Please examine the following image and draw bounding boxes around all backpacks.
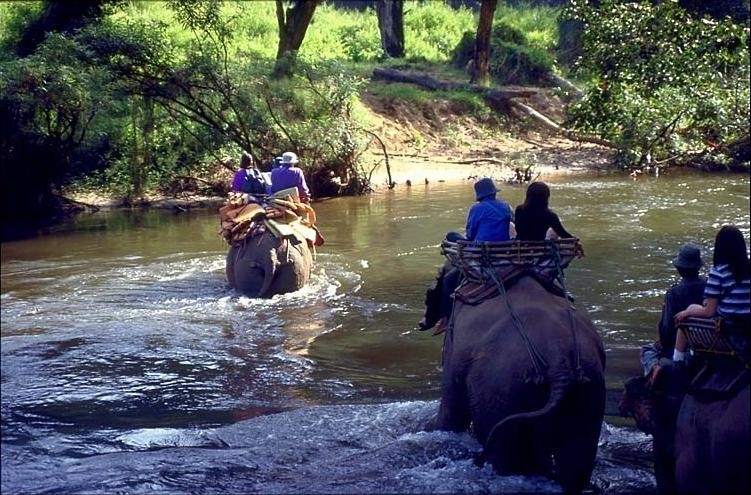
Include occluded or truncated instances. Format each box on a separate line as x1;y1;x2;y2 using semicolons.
243;167;267;195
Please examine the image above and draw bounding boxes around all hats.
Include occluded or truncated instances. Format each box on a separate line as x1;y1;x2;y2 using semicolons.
271;155;283;168
280;152;300;165
672;244;705;270
472;177;501;201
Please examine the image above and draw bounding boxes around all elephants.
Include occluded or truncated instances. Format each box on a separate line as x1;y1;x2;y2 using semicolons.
618;354;751;494
435;275;608;495
225;220;311;299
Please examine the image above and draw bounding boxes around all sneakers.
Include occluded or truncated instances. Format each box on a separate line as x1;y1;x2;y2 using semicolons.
648;364;667;400
670;359;688;378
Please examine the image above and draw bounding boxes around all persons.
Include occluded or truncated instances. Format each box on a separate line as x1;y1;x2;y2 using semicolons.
512;181;588;303
417;176;511;336
266;152;312;205
272;156;283;168
639;243;709;397
232;152;265;194
657;225;750;372
